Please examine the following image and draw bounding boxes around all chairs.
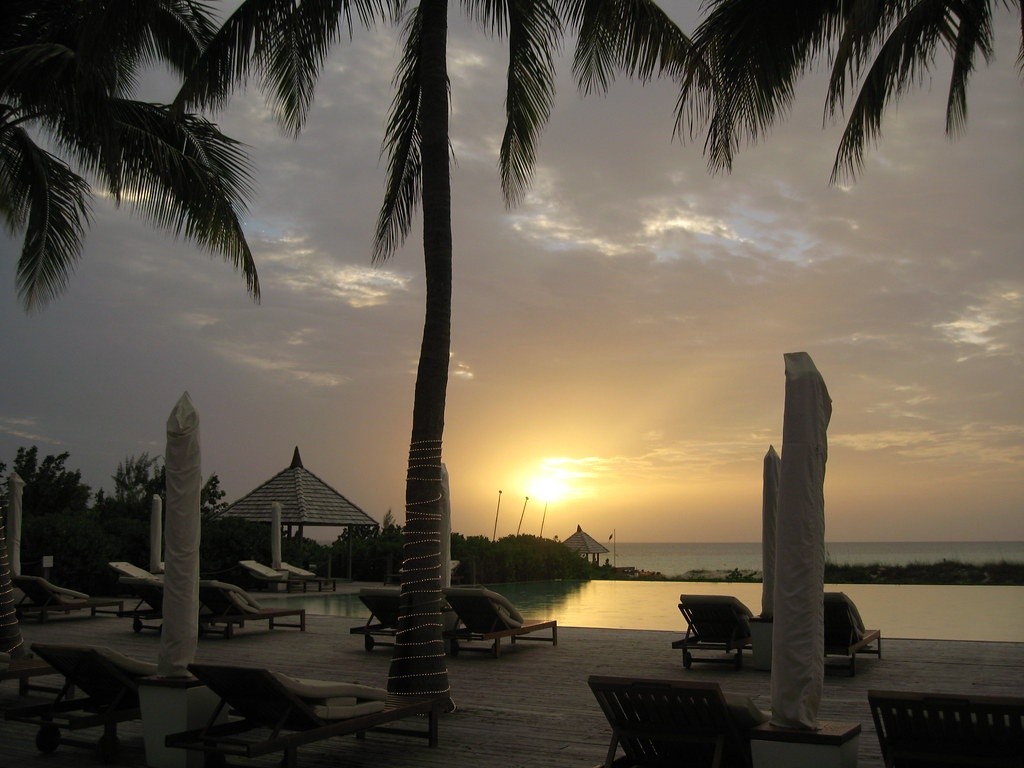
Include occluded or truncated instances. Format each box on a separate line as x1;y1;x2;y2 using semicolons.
239;560;306;593
118;577;162;634
4;643;159;761
672;594;754;668
165;664;438;768
825;592;882;676
349;589;466;656
199;580;306;638
13;576;124;623
587;675;772;768
867;689;1024;768
281;562;336;592
442;588;557;657
